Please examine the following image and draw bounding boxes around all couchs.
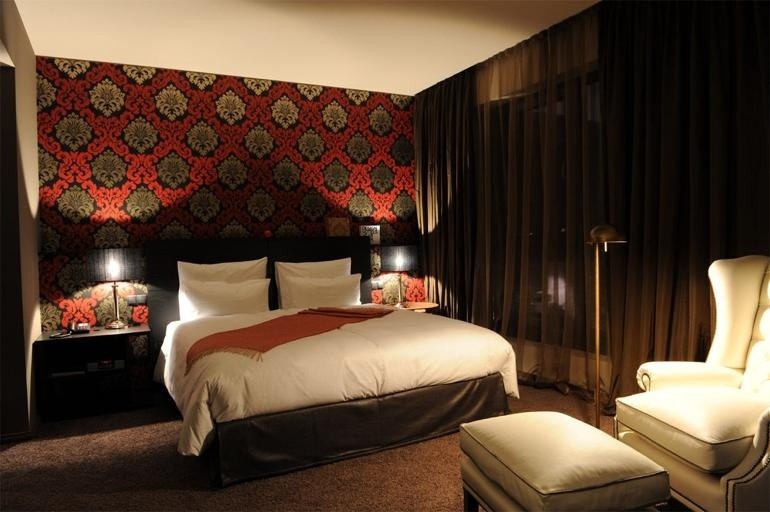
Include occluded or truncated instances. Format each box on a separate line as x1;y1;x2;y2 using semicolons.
611;252;770;512
456;410;672;512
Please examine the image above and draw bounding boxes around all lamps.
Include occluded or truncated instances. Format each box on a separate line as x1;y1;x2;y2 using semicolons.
378;244;422;309
584;223;631;431
85;247;149;329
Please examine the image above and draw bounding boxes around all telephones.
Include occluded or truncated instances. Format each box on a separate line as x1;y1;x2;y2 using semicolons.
67;321;90;334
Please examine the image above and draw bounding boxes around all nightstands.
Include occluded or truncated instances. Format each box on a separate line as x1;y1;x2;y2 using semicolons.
32;324;152;414
385;301;439;313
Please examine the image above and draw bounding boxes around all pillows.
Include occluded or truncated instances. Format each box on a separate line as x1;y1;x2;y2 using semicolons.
281;273;363;312
175;255;269;290
178;277;272;322
273;257;352;310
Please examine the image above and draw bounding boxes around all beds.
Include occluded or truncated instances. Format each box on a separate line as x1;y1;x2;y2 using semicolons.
146;235;523;491
